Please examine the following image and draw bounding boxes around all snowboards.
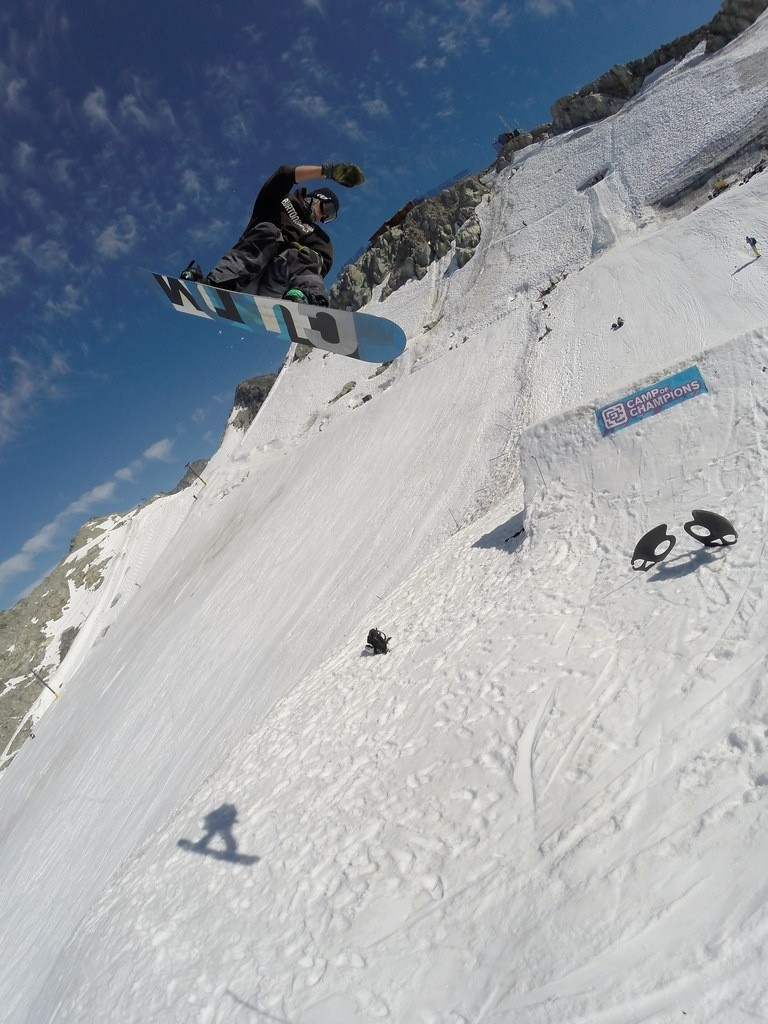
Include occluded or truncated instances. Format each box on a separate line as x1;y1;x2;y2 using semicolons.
136;265;408;367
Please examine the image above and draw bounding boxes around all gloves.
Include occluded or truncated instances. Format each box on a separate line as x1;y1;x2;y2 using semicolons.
321;162;365;188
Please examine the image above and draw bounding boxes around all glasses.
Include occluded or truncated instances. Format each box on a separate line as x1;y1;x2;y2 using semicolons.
320;201;337;224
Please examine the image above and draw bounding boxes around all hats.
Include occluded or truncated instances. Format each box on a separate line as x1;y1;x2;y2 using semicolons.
305;187;340;211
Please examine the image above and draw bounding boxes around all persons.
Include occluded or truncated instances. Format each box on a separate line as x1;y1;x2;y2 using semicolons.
180;162;365;308
612;317;624;328
746;236;761;259
